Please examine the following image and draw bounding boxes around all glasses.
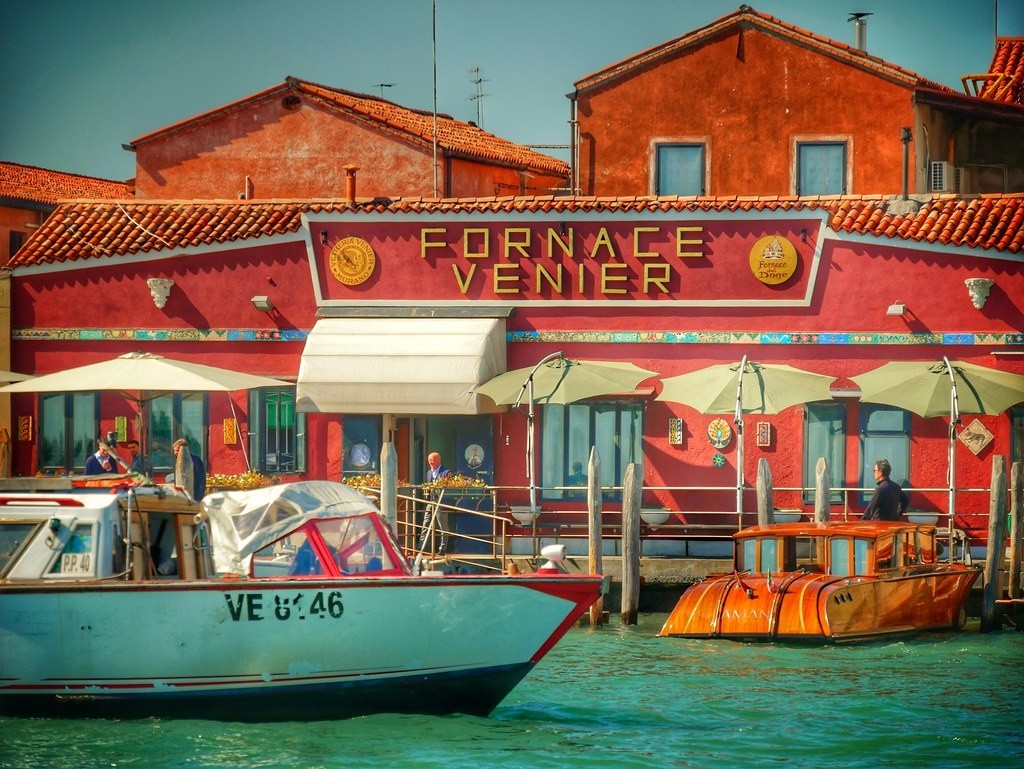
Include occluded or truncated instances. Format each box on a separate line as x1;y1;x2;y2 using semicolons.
873;469;879;472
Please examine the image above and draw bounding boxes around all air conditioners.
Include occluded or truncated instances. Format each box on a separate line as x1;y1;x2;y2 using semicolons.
954;167;973;194
932;161;955;194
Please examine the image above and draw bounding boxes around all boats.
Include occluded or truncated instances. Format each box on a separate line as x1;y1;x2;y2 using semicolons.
2;480;614;720
660;521;987;646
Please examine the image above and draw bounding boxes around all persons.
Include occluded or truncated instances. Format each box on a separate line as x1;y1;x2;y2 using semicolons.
417;453;453;557
171;437;205;501
859;459;909;522
86;440;154;478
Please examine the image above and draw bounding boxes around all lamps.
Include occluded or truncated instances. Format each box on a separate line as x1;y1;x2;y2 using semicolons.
252;296;273;312
887;299;906;318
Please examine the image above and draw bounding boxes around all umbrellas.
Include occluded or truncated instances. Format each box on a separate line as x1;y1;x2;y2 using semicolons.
473;354;659;557
655;358;839;512
846;358;1024;531
0;356;295;472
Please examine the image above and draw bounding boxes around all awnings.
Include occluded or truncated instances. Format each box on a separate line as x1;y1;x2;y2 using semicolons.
291;315;506;415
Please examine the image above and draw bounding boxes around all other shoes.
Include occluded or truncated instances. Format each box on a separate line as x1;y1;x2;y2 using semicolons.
408;548;424;555
437;550;445;555
